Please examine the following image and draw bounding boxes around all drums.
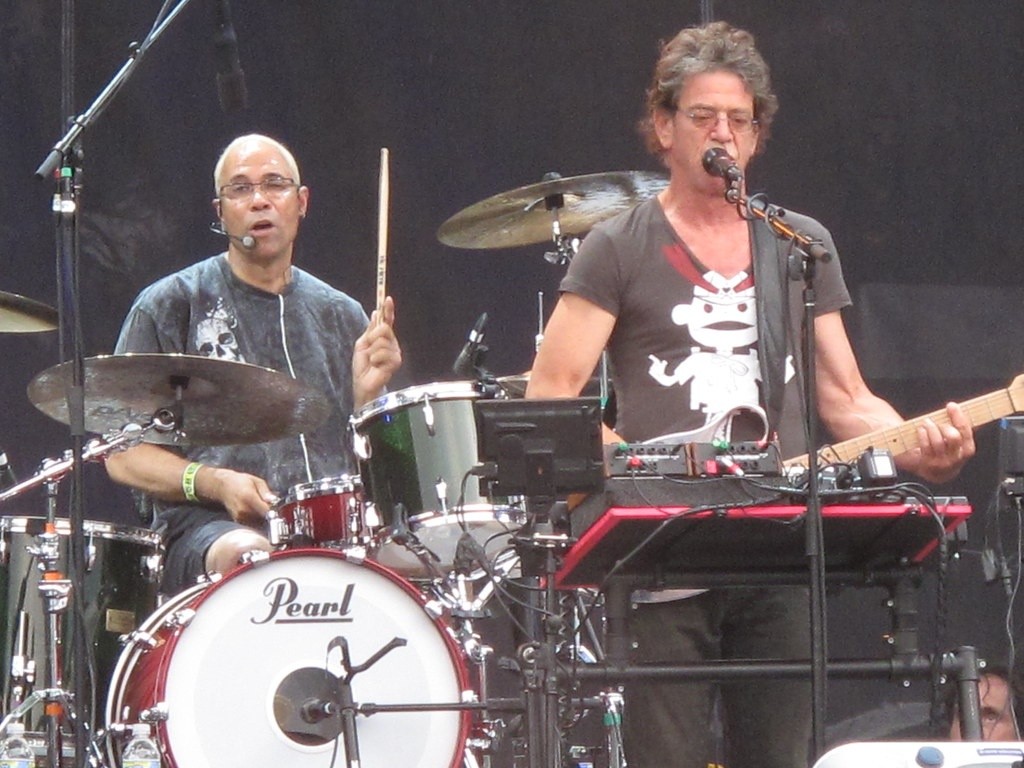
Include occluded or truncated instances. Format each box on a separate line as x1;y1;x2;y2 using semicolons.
263;474;377;551
101;546;481;768
344;368;535;573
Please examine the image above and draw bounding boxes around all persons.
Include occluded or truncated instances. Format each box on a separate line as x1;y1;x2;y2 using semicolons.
527;23;976;768
100;134;402;594
941;664;1024;743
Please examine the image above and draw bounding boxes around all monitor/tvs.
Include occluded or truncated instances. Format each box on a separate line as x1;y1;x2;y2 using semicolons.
475;399;604;496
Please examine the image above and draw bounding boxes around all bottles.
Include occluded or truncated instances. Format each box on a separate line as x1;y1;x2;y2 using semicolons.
122;724;160;768
0;723;35;768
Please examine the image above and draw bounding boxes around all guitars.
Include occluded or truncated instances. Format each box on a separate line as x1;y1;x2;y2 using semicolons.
553;370;1024;522
1;513;168;759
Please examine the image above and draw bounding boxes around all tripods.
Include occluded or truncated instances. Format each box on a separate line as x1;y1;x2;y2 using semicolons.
0;380;184;768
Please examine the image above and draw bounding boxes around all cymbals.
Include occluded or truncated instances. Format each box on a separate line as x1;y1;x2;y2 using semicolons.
436;168;671;253
1;288;68;338
25;351;330;447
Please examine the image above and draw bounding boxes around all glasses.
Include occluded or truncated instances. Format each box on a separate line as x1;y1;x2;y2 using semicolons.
218;177;300;200
671;105;760;132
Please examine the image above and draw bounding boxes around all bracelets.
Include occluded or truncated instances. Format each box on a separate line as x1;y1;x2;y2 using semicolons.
181;462;207;505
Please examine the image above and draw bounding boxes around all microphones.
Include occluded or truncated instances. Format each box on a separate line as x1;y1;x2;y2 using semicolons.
209;222;255;248
204;0;249;114
299;697;336;723
453;312;488;377
702;148;742;181
